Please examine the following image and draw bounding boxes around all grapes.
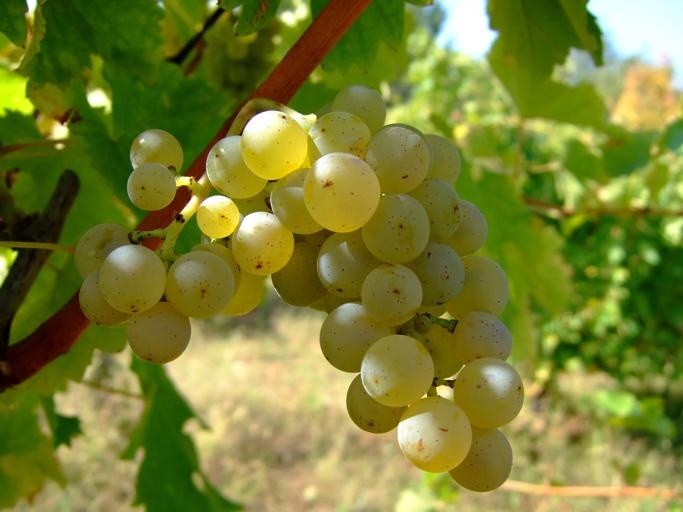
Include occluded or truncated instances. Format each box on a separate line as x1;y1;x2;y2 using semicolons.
74;83;525;493
